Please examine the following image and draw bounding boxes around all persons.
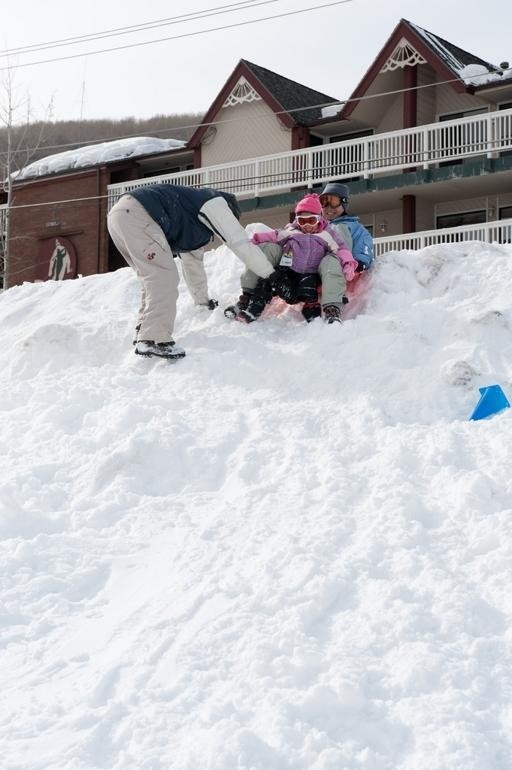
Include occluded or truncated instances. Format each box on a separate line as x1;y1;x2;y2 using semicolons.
106;182;279;361
224;181;376;324
235;192;358;324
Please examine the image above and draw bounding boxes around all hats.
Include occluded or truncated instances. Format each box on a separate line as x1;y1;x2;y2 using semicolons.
295;192;322;215
319;182;350;199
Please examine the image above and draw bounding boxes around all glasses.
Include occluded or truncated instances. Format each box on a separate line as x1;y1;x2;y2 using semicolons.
319;197;349;209
295;213;321;227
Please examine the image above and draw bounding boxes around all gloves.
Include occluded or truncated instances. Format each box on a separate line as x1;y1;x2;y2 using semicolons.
341;263;356;282
266;269;297;305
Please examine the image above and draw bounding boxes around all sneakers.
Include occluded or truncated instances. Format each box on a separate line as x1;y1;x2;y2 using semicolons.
132;324;186;360
223;293;266;325
301;302;344;326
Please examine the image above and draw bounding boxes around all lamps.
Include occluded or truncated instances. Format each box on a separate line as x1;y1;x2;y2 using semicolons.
380;219;389;233
487;204;495;216
